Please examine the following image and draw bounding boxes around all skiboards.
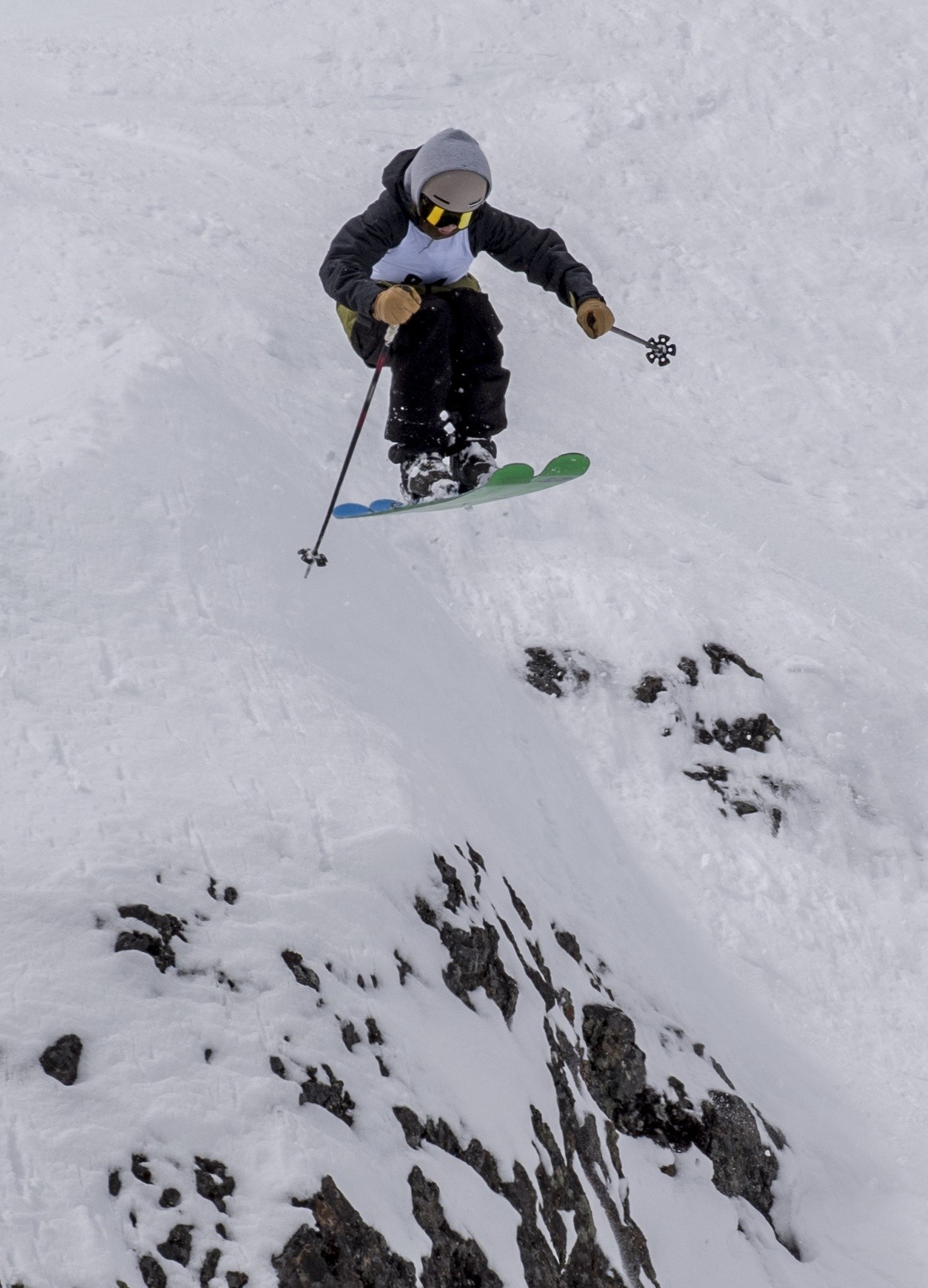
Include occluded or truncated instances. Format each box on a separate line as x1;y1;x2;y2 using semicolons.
331;452;592;521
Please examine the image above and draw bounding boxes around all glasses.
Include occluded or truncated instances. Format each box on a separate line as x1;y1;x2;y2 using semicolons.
419;199;477;230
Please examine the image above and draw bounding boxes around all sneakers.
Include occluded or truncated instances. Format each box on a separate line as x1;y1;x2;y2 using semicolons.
400;448;460;506
452;441;498;493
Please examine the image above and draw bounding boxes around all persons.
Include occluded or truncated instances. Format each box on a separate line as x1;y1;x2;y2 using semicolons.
319;127;615;503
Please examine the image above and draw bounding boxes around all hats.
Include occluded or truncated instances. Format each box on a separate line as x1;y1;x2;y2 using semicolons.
420;169;489;211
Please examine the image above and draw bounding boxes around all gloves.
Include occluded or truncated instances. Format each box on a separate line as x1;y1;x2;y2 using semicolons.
371;284;421;325
576;297;614;339
